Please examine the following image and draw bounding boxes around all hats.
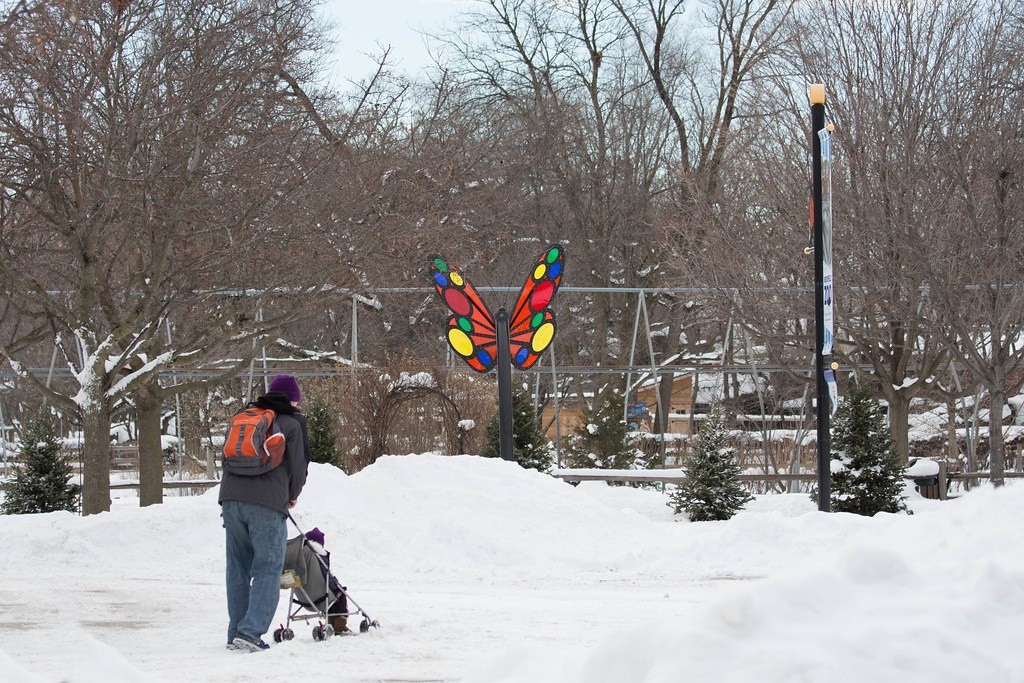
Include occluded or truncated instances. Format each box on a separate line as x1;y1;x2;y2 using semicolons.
306;527;325;546
268;374;300;402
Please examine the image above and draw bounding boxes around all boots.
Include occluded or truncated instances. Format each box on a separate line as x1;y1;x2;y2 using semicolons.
328;616;333;625
333;616;352;636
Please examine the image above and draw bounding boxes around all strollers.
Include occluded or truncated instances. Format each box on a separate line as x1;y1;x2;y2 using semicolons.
274;504;381;644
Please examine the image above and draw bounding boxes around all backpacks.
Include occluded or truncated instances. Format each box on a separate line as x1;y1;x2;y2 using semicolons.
221;404;286;476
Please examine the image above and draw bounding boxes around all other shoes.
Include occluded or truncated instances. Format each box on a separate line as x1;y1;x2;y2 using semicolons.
233;631;270;652
226;640;239;650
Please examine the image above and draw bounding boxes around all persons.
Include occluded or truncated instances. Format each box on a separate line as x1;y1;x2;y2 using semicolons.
217;374;311;652
305;527;358;636
